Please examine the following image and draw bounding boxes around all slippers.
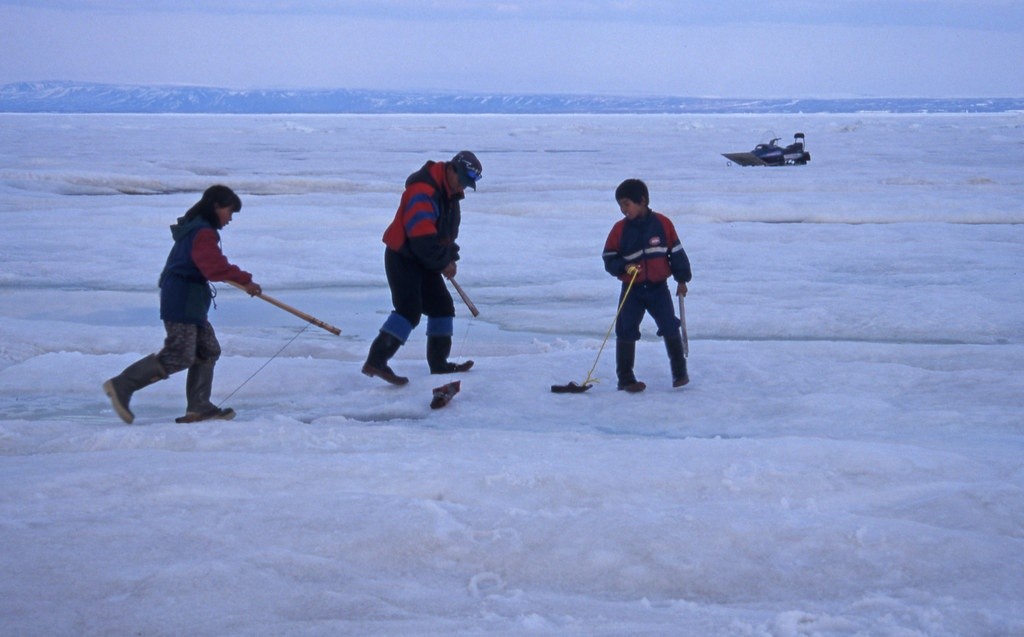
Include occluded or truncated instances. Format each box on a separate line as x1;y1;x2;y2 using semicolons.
431;381;461;410
551;381;592;394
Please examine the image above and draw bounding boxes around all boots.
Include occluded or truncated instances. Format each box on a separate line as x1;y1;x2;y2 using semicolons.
662;329;689;387
104;353;169;424
426;316;474;374
616;337;646;392
362;311;409;385
186;357;237;422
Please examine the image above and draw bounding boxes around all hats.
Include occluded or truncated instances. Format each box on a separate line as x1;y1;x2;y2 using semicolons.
452;151;484;191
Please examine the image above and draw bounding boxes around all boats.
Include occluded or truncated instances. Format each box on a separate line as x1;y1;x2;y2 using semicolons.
720;138;810;167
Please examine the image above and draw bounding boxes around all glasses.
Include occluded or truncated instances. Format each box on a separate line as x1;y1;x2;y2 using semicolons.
455;154;483;182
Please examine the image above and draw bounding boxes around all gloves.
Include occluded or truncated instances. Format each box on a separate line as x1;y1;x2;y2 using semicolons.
246;283;262;297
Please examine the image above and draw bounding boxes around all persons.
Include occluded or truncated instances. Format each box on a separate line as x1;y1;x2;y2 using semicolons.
362;149;484;386
103;185;263;423
602;178;692;392
